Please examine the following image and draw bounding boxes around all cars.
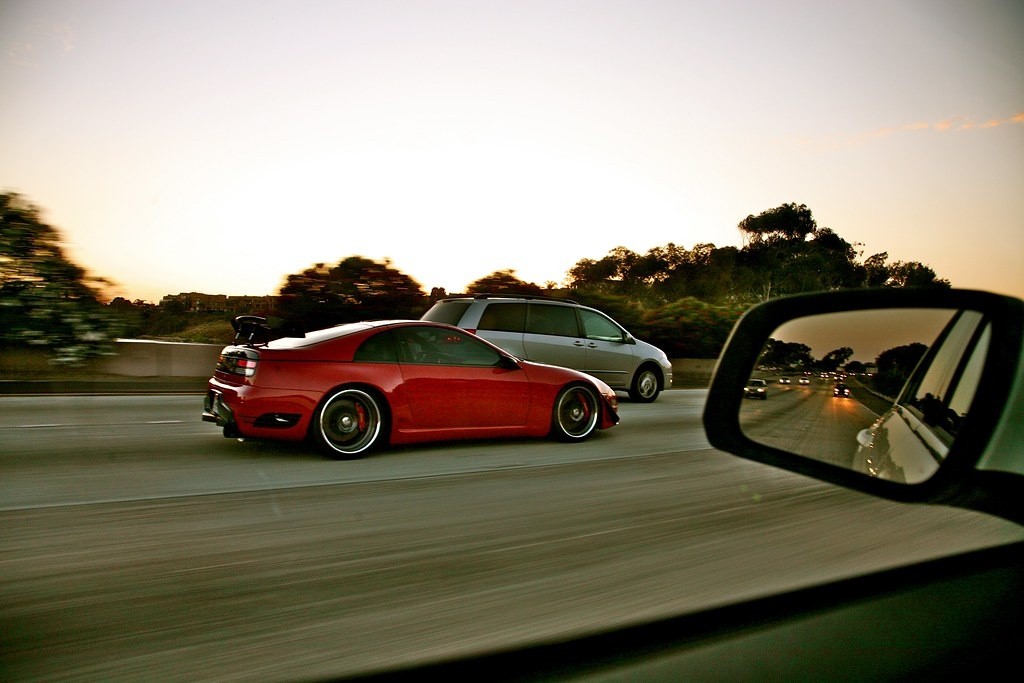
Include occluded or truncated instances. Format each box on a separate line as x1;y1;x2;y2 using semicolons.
739;368;876;408
199;313;620;455
414;294;676;405
